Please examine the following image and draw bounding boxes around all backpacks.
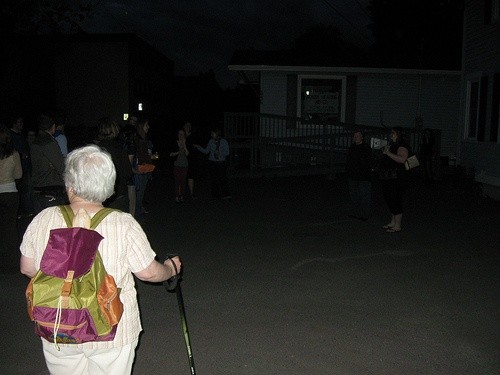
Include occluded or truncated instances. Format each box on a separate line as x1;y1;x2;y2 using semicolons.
25;204;123;344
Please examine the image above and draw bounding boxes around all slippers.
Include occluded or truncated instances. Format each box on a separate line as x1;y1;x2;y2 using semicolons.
384;223;405;235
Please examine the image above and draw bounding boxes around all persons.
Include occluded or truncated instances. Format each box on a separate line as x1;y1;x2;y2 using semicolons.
0;115;231;273
19;145;182;375
345;126;436;232
